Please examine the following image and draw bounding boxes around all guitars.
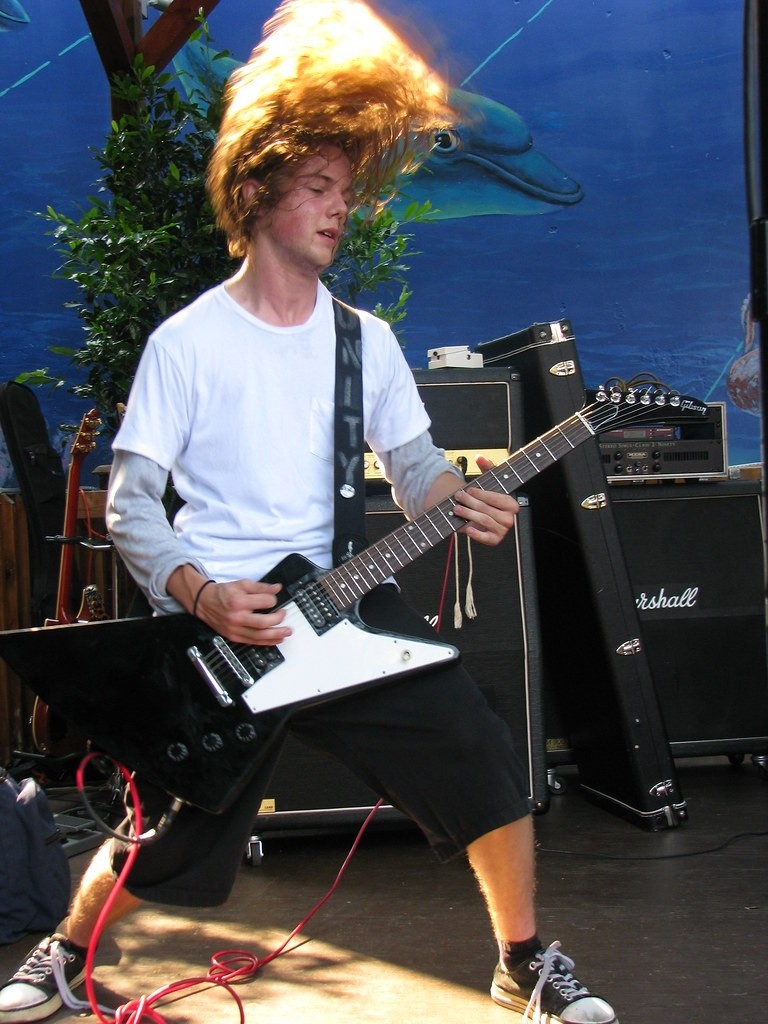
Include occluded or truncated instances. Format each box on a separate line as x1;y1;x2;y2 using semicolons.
0;386;715;822
30;404;102;753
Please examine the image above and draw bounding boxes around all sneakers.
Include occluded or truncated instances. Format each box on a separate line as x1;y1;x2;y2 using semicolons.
0;936;87;1024
491;941;620;1024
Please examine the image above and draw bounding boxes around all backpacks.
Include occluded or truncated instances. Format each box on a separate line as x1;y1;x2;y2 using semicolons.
1;767;71;942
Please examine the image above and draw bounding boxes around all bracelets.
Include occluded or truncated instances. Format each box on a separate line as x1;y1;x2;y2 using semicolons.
193;579;218;616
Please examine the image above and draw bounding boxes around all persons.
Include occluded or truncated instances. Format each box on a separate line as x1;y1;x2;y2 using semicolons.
0;38;627;1024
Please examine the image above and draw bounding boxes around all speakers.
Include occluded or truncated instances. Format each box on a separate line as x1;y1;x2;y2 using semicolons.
250;491;548;833
608;480;768;755
